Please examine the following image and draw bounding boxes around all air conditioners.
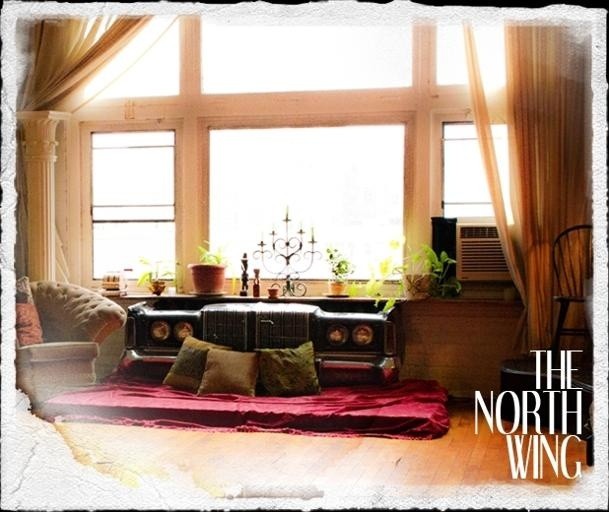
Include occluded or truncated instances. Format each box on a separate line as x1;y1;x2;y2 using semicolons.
455;215;516;300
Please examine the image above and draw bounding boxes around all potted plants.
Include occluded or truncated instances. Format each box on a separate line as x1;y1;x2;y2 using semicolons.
136;256;177;295
324;244;352;298
370;236;463;313
187;238;230;297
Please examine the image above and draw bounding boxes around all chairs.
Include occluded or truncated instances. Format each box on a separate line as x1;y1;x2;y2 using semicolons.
499;222;593;467
16;281;129;406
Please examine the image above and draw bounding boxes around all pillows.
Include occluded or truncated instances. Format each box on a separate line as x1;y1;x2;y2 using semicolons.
16;276;43;346
197;347;260;397
162;335;233;393
252;340;322;397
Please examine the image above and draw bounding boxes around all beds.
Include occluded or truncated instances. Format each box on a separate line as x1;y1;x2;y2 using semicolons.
42;302;451;440
